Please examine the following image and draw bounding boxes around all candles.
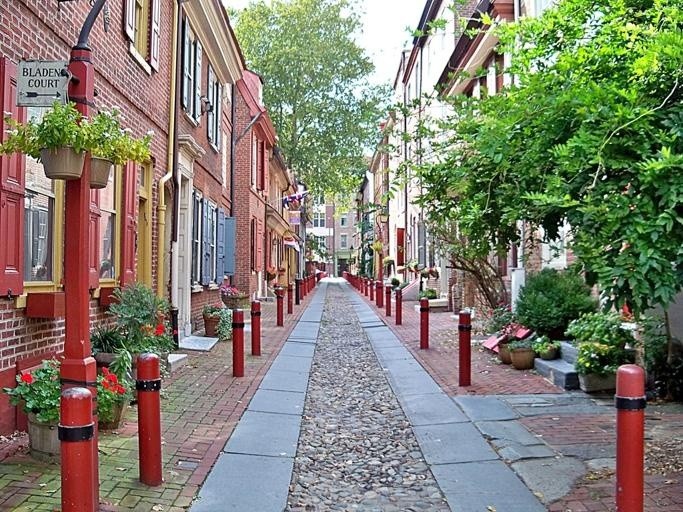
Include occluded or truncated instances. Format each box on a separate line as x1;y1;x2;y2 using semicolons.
0;99;91;181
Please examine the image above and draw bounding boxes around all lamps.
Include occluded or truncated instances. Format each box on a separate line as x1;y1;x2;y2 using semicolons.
376;206;390;237
197;95;213;119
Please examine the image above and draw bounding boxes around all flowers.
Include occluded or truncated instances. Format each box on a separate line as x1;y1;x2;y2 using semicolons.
2;354;65;425
481;304;518;343
574;341;633;378
139;306;178;353
217;284;239;296
96;366;136;423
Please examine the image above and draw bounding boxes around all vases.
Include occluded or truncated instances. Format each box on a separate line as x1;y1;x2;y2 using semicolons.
498;341;513;364
221;294;250;309
26;411;62;465
153;350;169;372
577;373;616;392
98;396;124;430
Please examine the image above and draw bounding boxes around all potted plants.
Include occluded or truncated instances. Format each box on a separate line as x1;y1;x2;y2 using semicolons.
203;303;234;341
534;335;560;360
88;319;139;384
88;105;154;189
508;339;536;370
